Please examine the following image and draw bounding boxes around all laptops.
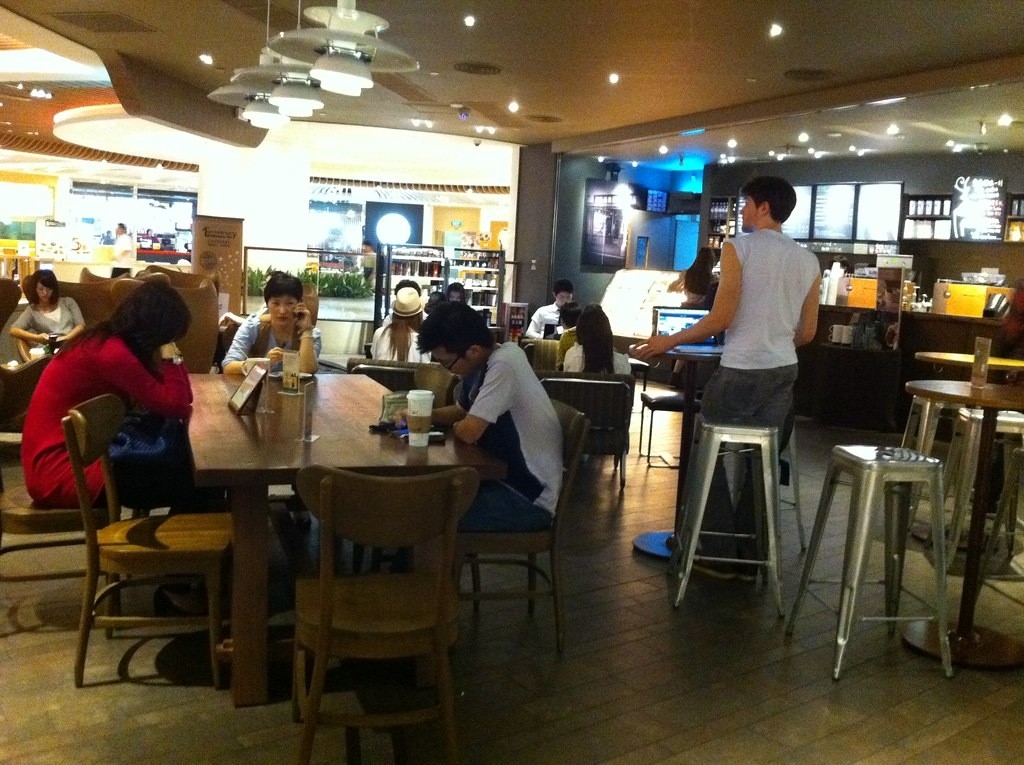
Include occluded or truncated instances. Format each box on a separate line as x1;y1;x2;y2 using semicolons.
653;306;725;353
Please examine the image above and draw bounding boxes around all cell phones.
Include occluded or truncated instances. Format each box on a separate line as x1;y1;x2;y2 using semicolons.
396;432;443;442
49;334;58;341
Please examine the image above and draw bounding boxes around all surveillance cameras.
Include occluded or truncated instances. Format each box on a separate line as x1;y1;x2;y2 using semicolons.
474;140;481;146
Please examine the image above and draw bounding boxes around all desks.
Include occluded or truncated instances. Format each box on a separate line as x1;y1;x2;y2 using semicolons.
182;372;507;707
814;341;901;433
911;352;1024;552
901;379;1024;672
629;342;725;558
629;357;650;456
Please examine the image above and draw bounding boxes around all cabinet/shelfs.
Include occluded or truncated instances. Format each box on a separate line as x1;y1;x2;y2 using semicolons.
897;194;1024;243
706;196;738;269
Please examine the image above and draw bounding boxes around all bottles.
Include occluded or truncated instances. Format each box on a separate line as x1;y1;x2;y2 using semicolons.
455;251;499;268
511;309;524;325
718;203;728;220
464;289;496;306
855;314;897;350
477;309;491;327
711;203;718;220
391;260;405;275
509;329;523;344
713;221;720;234
406;260;440;277
421;280;443;299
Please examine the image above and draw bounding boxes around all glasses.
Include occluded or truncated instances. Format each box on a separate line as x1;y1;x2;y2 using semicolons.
432;343;476;372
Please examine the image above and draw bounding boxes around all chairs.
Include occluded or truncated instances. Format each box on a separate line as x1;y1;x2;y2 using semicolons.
0;264;636;765
639;357;703;469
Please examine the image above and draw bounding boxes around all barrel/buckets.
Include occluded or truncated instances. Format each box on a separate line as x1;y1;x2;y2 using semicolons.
984;294;1011;318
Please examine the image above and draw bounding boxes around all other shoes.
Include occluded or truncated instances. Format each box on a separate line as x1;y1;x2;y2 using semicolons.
738;559;759;580
692;548;736;579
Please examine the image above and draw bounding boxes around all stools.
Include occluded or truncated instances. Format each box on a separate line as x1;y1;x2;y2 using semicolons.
901;397;1024;580
786;443;954;683
666;413;783;617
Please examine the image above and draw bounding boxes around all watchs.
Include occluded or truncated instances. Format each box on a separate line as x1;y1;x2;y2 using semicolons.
162;354;182;366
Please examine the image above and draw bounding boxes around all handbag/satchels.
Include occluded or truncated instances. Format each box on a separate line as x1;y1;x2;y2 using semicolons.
109;410;191;509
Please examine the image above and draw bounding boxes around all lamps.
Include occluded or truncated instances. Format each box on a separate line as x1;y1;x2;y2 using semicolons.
206;0;420;130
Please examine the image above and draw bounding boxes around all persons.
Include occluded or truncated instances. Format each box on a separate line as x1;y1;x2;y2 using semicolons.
145;229;160;243
369;279;468;365
21;279;232;619
666;246;719;388
111;223;136;278
555;302;582;370
220;271;319;532
526;278;574;339
392;299;561;575
10;268;86;351
564;301;633;377
633;178;821;580
360;241;376;284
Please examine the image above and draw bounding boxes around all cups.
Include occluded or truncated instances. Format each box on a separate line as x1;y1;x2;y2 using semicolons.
449;278;472;287
30;348;45;360
828;324;853;347
242;358;270;388
473;280;497;287
406;390;434;446
393;248;443;258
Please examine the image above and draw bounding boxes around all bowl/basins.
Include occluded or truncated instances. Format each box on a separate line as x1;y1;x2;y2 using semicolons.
854;263;868;275
962;273;1005;287
864;268;878;278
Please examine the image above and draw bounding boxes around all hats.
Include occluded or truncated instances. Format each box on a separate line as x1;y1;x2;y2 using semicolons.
391;287;425;316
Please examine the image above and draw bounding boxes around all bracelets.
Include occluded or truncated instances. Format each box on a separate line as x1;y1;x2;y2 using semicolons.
297;325;314;335
299;335;315;341
